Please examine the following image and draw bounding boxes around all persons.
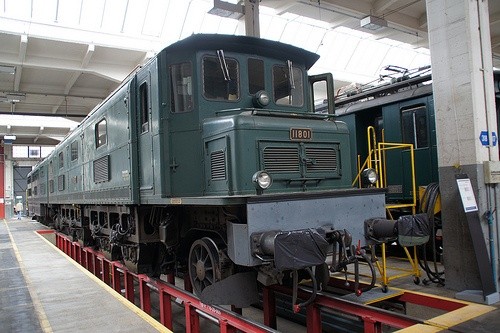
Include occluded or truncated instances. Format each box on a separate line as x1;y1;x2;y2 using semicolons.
15;200;23;220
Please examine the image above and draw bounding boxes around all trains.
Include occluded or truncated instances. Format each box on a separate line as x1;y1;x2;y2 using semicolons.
352;300;390;307
24;32;343;299
312;65;443;262
